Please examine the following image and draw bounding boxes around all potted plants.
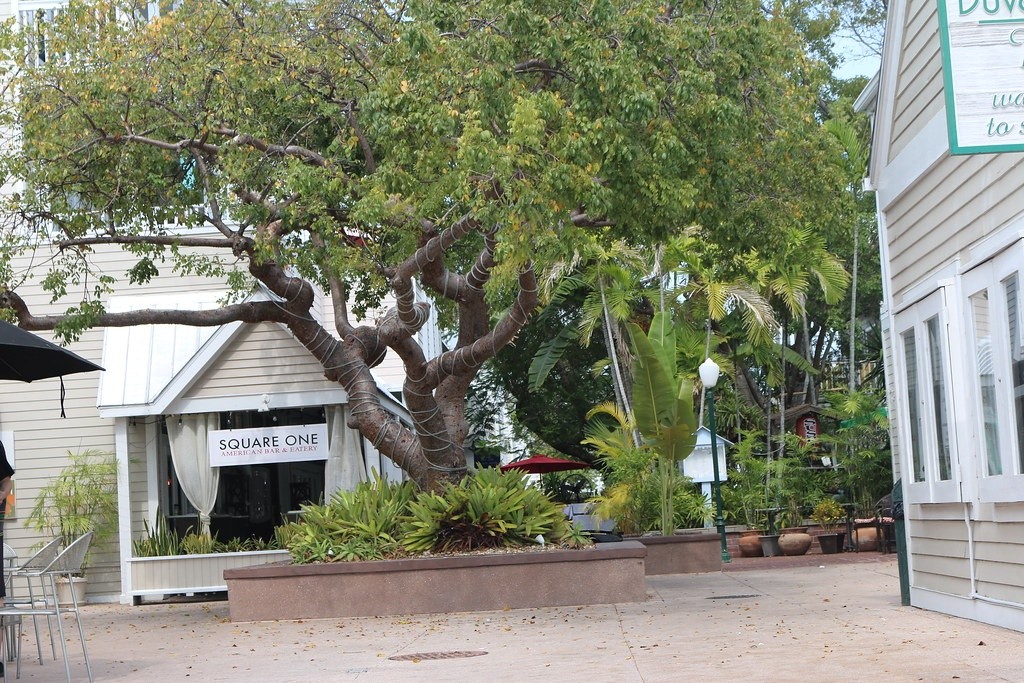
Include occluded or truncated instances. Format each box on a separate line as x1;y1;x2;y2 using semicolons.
810;498;846;554
729;429;831;556
24;443;120;610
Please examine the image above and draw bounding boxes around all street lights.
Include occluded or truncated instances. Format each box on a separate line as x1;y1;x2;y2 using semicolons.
698;358;732;562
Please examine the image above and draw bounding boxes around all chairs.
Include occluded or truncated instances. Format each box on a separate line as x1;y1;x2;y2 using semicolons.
853;494;894;554
0;530;93;683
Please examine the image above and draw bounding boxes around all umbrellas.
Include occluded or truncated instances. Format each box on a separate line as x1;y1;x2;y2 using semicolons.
500;454;590;489
0;318;106;419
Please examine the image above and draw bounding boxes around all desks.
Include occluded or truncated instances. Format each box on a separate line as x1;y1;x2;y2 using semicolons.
755;502;857;552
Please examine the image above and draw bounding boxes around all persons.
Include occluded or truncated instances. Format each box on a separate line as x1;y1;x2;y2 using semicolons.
0;437;15;677
